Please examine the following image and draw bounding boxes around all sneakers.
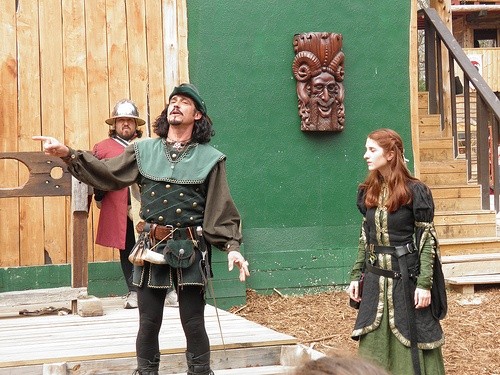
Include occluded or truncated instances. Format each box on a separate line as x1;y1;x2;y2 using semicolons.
164;290;180;307
123;290;137;308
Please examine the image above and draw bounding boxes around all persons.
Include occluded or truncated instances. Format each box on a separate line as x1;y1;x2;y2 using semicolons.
348;129;447;375
91;100;179;309
293;350;390;375
32;83;250;375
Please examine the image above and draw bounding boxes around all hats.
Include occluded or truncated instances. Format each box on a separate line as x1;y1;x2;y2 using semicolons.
169;83;207;115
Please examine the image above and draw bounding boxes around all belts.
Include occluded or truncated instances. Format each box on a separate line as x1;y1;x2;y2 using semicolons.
366;244;421;375
136;221;203;241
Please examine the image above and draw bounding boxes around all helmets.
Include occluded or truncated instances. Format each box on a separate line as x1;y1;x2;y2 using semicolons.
105;99;146;126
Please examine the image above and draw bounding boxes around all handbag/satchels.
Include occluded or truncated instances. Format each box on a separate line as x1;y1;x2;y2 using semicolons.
163;238;196;268
350;275;365;309
128;236;145;267
